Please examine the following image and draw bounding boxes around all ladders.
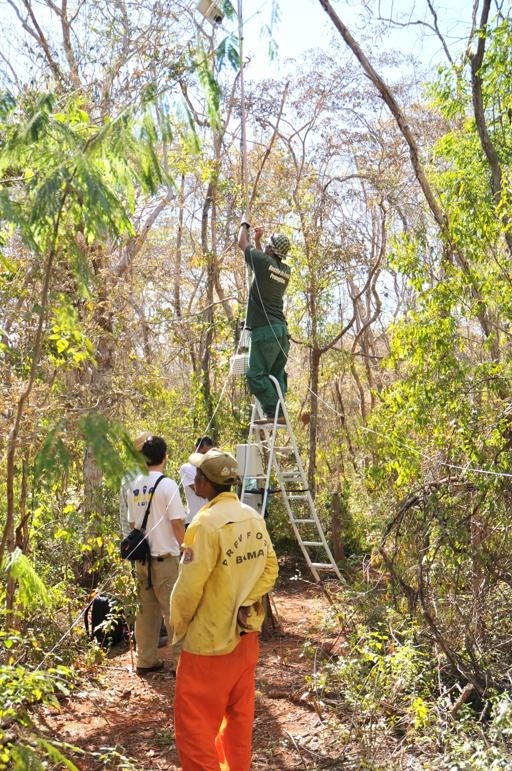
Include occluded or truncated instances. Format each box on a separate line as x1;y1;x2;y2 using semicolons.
239;376;343;583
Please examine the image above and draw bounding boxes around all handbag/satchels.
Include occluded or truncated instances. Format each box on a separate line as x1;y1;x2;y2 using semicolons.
121;528;150;562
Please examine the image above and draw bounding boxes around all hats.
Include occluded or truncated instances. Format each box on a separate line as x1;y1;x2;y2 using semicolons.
132;431;152;451
187;451;240;485
268;233;291;261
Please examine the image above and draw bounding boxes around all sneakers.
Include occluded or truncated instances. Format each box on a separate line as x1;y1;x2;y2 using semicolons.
254;416;288;425
137;661;165;676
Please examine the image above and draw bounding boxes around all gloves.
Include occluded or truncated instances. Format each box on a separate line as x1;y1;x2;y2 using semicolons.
240;212;250;229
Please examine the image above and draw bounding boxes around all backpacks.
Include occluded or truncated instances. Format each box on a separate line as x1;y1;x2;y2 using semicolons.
83;593;132;649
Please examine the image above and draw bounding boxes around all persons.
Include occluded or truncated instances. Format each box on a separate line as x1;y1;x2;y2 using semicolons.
126;436;185;679
168;448;280;771
119;432;167;649
236;213;292;423
180;438;212;530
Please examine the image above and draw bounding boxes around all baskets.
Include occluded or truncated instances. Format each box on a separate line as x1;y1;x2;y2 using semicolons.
229;353;249;376
